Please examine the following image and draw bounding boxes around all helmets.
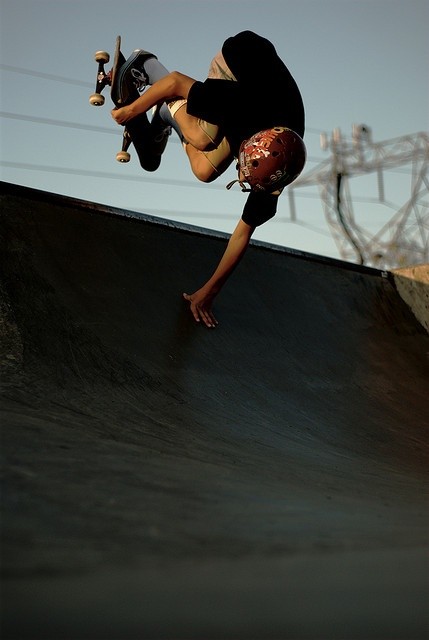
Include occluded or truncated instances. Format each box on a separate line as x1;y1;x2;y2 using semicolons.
237;126;306;193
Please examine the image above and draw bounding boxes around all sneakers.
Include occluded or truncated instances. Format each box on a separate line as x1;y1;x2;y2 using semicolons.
116;49;158;104
146;95;184;156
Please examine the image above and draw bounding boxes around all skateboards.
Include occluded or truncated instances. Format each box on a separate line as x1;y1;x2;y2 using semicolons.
87;34;160;172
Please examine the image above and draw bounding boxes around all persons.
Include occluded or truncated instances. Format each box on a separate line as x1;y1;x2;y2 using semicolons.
111;30;306;329
